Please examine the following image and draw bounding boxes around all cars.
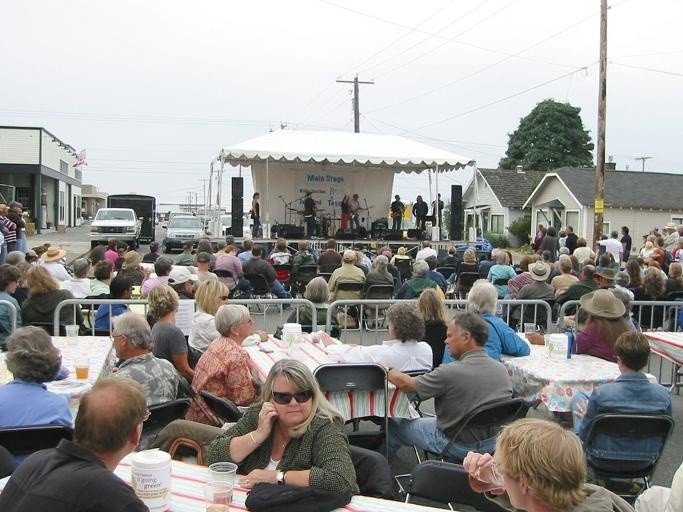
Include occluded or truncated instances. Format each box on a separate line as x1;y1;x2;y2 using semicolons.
197;207;253;237
154;216;158;225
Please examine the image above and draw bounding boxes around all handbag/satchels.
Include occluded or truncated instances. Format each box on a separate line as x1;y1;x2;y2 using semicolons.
244;481;345;512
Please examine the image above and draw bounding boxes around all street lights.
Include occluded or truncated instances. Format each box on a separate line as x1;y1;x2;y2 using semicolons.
607;155;613;162
624;164;629;170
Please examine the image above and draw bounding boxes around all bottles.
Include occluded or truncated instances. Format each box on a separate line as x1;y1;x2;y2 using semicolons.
282;323;301;343
565;326;573;359
542;334;550;358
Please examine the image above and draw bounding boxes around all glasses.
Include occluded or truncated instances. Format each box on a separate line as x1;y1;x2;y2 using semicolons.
271;388;312;404
109;334;122;341
220;296;228;301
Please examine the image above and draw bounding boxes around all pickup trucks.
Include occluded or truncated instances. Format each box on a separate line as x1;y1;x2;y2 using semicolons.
86;207;143;250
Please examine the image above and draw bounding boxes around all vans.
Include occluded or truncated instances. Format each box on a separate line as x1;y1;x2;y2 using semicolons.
167;211;195;227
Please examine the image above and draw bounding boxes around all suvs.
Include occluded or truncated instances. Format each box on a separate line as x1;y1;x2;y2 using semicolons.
161;214;207;253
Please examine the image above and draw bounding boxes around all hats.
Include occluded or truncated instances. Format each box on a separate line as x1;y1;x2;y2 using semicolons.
528;261;551;281
343;250;355;261
26;250;38;258
666;223;676;228
580;289;626;319
168;267;198;286
596;268;616;281
121;251;143;268
41;246;66;262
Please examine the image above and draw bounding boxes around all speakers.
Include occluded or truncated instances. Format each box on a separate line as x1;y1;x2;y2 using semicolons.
407;229;420;238
335;228;355;241
231;176;243;237
281;227;303;238
383;230;402;240
451;185;461;240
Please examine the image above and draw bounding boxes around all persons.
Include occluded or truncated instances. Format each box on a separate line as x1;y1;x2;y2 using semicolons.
1;189;683;512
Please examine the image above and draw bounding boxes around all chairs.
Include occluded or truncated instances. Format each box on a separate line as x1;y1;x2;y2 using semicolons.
0;238;682;512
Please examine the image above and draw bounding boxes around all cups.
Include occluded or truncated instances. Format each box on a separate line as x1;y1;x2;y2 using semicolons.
74;356;90;381
202;459;238;512
65;323;79;345
548;319;561;334
523;322;539;337
468;457;502;494
